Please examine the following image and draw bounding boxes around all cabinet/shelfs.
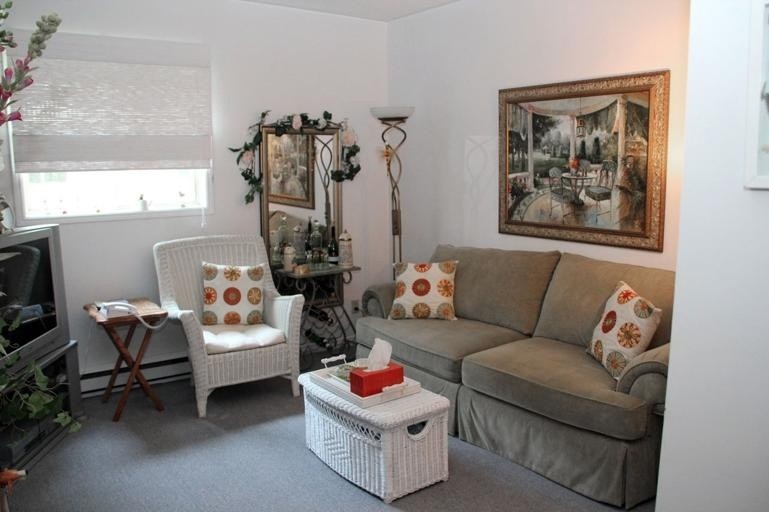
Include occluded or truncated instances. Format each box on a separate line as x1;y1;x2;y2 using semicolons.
0;336;88;481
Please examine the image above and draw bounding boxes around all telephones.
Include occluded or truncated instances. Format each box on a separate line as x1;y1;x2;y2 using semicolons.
91;298;138;320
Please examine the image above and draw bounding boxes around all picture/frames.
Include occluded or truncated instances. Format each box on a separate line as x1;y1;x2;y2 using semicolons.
498;67;671;253
746;0;769;191
267;132;317;211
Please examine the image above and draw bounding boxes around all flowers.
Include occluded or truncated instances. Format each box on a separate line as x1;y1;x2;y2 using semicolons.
0;1;63;147
507;174;527;199
239;106;362;204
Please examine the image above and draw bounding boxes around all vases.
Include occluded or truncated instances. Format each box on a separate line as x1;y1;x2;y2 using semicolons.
0;152;17;236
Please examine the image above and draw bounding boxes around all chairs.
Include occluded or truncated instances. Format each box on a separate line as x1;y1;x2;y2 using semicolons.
545;157;618;226
152;234;305;419
0;245;42;330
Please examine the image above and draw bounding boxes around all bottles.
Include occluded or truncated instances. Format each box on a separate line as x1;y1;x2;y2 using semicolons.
271;215;312;263
281;218;353;273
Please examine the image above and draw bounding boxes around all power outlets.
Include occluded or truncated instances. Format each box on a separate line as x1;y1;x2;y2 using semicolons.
351;299;360;314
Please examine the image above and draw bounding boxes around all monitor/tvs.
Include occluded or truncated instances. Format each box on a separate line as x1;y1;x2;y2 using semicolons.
0;224;69;382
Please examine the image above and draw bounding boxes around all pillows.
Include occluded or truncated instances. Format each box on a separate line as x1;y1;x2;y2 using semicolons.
584;274;665;387
200;258;269;324
388;258;460;324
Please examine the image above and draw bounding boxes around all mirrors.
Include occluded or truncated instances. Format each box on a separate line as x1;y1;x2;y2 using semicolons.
259;126;342;269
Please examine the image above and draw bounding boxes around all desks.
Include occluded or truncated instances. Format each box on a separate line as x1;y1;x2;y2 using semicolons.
278;259;363;368
85;298;169;422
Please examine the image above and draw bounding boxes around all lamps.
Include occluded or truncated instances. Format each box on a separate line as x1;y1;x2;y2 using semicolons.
370;105;416;279
576;97;588;137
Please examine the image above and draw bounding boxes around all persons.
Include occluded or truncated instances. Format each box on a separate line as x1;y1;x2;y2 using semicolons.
616;154;649;231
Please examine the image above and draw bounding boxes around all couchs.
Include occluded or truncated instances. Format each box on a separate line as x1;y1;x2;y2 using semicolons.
269;208;314;246
354;242;682;510
